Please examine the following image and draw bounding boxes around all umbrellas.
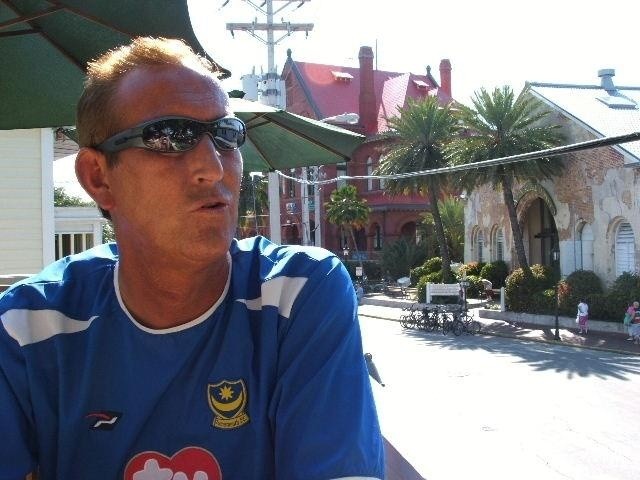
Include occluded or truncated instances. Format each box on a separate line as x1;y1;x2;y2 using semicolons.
0;0;233;134
223;89;367;175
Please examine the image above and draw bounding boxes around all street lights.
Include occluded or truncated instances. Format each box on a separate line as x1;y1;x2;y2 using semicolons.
343;244;350;267
550;243;563;342
300;112;361;246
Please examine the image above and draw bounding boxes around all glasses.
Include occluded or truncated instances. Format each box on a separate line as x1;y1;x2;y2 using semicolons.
96;114;246;158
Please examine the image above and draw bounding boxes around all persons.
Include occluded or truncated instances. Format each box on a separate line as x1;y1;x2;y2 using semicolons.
624;301;633;341
628;302;640;345
577;298;589;334
0;37;388;480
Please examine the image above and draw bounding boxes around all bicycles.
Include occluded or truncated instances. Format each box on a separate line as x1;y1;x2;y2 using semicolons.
399;301;482;337
